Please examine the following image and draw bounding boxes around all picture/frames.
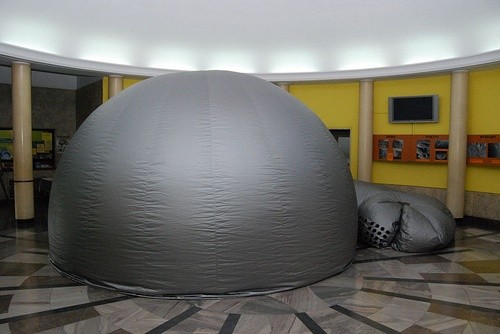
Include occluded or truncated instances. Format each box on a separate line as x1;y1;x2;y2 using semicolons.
0;126;57;172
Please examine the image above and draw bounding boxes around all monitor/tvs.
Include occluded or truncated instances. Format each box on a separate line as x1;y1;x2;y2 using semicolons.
388;94;439;123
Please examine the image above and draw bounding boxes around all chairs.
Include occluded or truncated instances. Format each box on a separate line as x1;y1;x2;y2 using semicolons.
0;177;16;228
